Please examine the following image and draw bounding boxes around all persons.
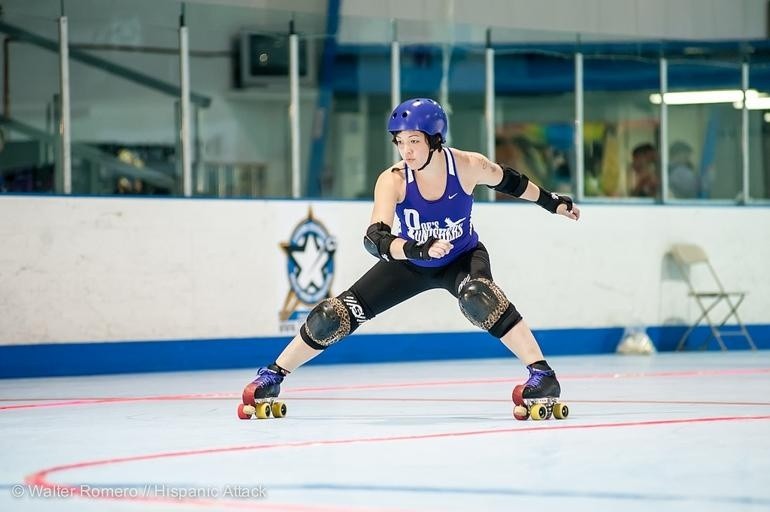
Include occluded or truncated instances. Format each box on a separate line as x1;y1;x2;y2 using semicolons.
245;98;579;405
669;139;705;198
632;142;660;200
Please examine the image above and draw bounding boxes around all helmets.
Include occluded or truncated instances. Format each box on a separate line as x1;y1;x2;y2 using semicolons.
387;98;447;143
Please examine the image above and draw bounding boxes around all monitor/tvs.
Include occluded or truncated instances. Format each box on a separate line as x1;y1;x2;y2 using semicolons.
232;26;313;92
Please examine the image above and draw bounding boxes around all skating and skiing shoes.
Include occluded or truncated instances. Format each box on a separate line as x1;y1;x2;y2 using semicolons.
238;365;287;419
512;366;569;420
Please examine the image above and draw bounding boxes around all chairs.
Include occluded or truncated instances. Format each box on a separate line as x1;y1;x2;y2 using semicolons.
668;242;757;351
